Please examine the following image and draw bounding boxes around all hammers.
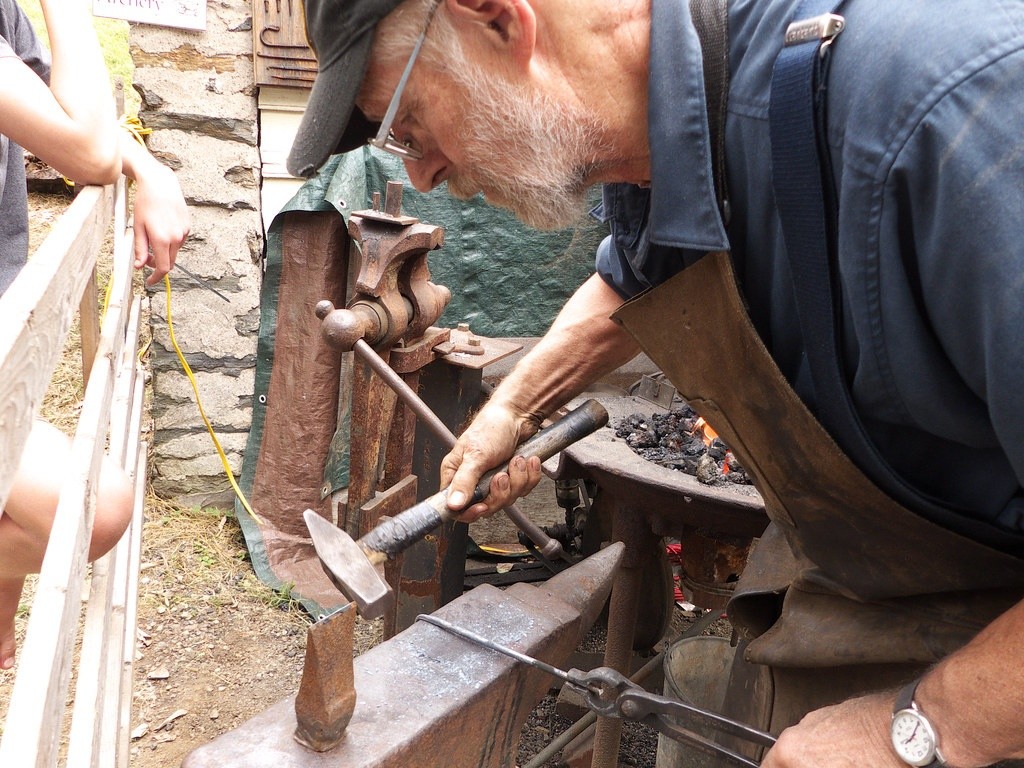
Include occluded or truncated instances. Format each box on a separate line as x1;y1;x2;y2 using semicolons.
304;400;610;620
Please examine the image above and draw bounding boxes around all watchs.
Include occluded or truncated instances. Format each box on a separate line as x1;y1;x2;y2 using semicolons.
891;679;945;768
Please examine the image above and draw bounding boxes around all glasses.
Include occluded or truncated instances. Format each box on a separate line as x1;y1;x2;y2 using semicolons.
367;1;443;161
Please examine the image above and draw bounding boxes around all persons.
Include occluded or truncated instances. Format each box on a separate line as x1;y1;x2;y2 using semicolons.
287;0;1024;768
0;0;212;647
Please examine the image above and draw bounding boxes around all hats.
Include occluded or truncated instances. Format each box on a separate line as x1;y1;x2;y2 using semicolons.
288;1;397;178
